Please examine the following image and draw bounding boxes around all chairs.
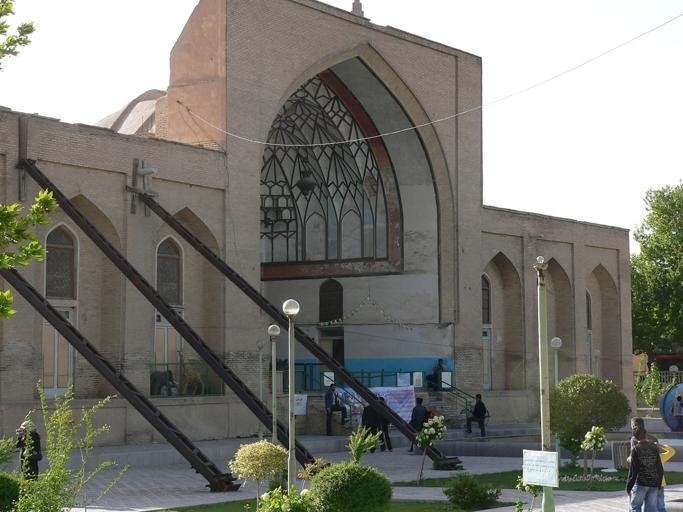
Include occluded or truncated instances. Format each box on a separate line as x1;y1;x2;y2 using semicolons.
150;359;208;397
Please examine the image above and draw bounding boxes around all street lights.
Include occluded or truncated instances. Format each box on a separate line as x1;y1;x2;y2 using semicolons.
550;336;562;387
257;338;264;442
534;255;555;511
282;299;300;497
268;324;280;490
669;365;679;385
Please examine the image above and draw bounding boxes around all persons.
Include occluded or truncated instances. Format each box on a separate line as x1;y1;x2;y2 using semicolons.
463;393;487;437
323;382;349;435
360;400;377;453
670;395;682;432
626;416;669;512
374;396;395;451
430;358;445;391
625;427;662;512
13;420;42;482
405;397;430;452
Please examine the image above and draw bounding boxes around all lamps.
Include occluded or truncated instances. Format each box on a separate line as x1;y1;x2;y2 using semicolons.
293;81;315;195
